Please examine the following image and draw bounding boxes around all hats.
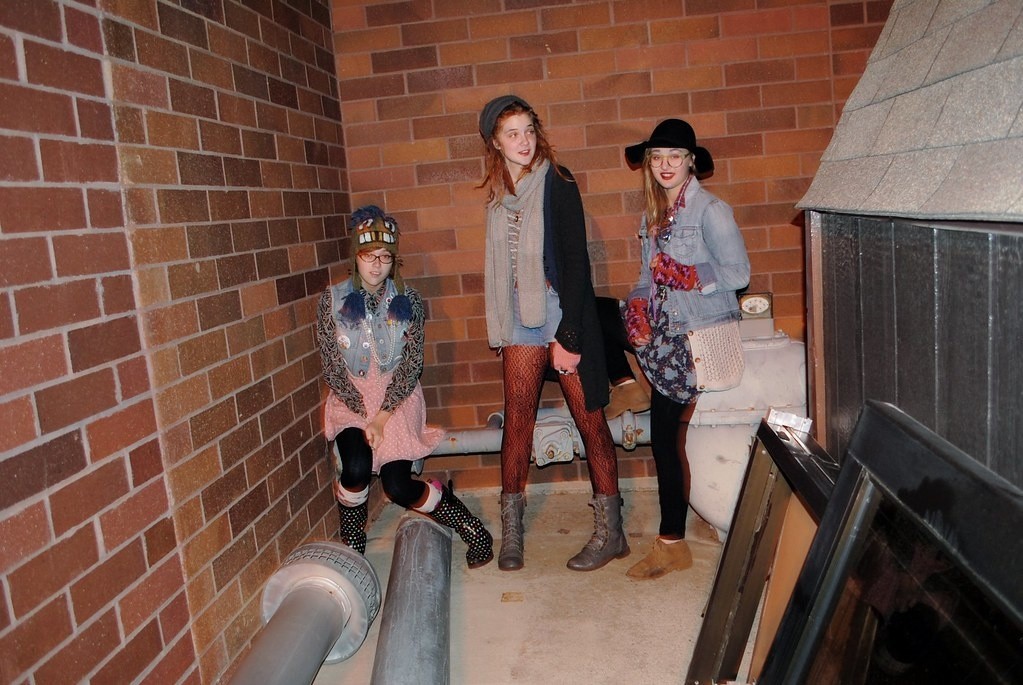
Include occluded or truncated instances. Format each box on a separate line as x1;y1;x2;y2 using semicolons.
624;119;715;181
337;203;412;324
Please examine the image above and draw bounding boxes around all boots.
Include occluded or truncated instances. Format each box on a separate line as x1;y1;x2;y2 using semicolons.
336;498;368;557
423;479;494;569
567;492;631;572
498;490;525;570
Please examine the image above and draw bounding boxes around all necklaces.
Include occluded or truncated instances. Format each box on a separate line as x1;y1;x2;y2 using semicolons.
361;318;396;366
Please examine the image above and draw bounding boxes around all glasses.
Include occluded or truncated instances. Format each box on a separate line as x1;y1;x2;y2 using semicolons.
646;152;691;168
356;253;394;264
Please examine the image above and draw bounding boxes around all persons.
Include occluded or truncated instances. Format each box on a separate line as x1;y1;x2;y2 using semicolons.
620;119;749;577
476;95;630;569
314;207;493;569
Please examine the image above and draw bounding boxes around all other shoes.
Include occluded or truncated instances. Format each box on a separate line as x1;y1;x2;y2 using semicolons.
626;538;692;579
602;381;650;420
